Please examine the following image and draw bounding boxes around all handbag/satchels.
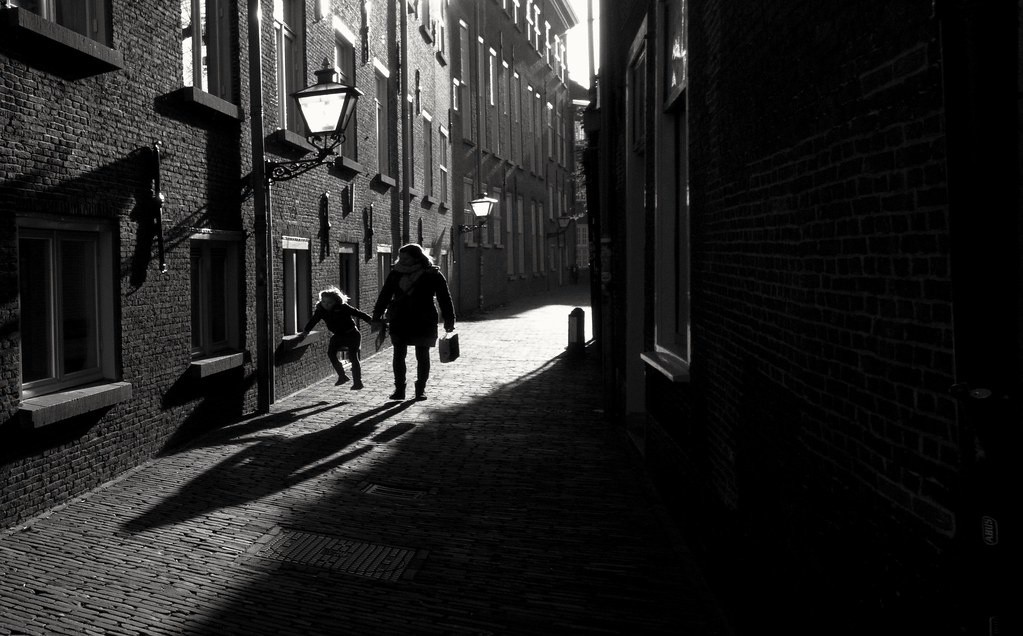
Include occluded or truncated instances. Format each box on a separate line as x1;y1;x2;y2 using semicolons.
439;329;460;363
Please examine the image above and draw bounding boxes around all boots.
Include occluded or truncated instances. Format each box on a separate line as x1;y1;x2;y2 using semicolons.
389;383;407;400
415;382;427;400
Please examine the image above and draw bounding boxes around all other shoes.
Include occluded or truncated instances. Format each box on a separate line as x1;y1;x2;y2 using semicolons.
334;374;350;386
350;379;364;390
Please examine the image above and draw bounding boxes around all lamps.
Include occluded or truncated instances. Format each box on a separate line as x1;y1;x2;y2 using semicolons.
547;212;570;239
457;193;499;235
265;57;364;188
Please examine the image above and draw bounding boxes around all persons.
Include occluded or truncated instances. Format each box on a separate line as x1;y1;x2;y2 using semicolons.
372;244;455;400
301;290;371;390
